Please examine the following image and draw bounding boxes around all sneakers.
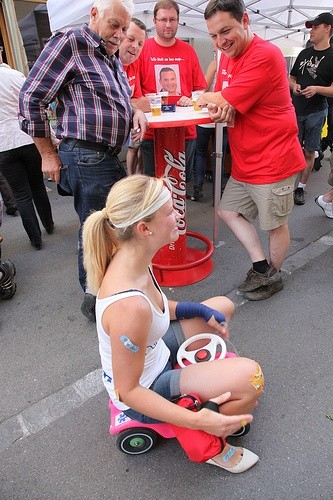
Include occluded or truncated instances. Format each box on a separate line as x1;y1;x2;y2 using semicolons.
294;187;307;204
243;278;284;301
0;258;17;300
315;195;333;218
238;265;281;291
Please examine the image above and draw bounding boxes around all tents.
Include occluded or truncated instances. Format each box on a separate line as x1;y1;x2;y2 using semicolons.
18;3;52;72
46;0;332;246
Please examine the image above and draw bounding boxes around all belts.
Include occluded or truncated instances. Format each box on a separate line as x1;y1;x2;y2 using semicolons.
63;138;123;157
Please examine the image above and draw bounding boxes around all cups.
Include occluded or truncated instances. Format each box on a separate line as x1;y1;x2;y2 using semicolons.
191;91;203;110
158;92;168;104
145;93;156;101
149;96;161;115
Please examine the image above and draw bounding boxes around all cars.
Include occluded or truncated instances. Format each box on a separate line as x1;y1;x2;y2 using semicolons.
106;333;255;456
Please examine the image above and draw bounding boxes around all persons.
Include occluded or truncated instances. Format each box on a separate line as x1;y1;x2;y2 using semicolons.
127;0;209;182
191;48;229;207
0;257;17;300
119;17;151;114
18;0;149;321
126;129;144;177
195;0;307;301
82;175;264;473
159;68;180;96
288;12;333;218
0;50;55;251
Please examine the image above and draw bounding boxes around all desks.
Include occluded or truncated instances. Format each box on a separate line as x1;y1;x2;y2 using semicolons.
131;107;214;287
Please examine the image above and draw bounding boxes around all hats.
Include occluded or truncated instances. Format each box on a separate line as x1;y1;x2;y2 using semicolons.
306;13;333;28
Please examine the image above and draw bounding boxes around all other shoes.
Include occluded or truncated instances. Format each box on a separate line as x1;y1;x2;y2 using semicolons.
30;241;41;249
81;294;96;322
6;207;19;215
46;221;54;234
191;184;203;201
314;151;324;170
0;237;3;243
